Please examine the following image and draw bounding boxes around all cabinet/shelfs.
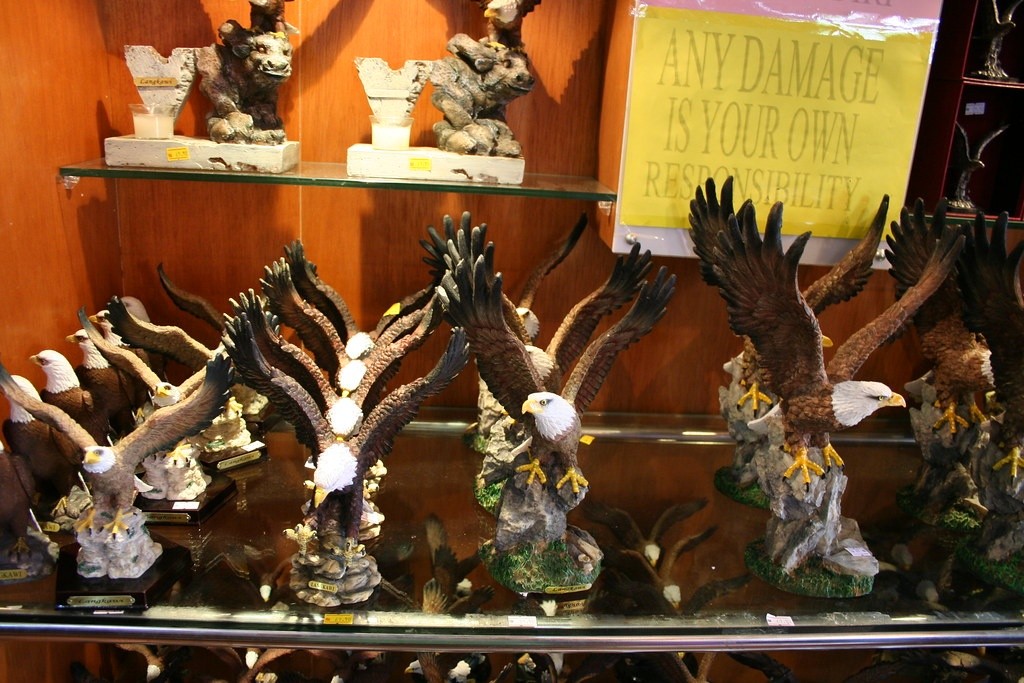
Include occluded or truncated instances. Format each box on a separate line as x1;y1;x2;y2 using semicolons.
2;0;1024;638
